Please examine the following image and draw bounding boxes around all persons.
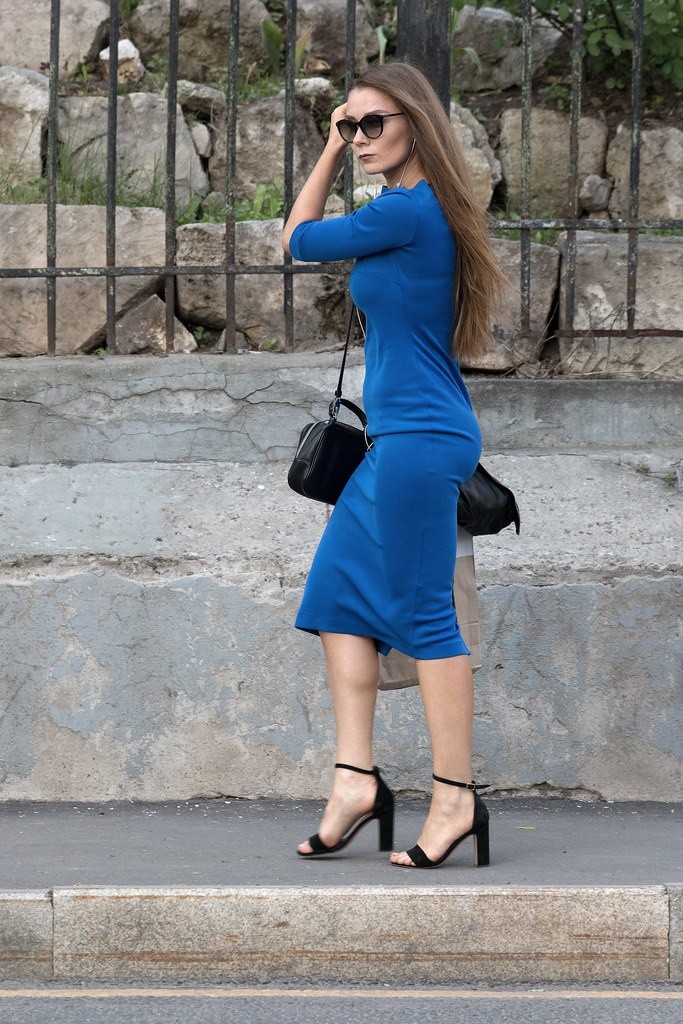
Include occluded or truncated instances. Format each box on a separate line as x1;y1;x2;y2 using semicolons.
283;60;491;869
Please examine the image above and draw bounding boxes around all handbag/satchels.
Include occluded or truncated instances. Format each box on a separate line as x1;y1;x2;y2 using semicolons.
286;397;374;504
371;526;482;690
456;460;523;540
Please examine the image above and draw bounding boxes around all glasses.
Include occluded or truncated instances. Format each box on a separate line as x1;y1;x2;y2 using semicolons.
335;112;405;143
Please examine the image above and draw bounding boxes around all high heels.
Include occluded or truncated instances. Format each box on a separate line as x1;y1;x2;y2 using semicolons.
294;761;394;855
388;773;491;871
